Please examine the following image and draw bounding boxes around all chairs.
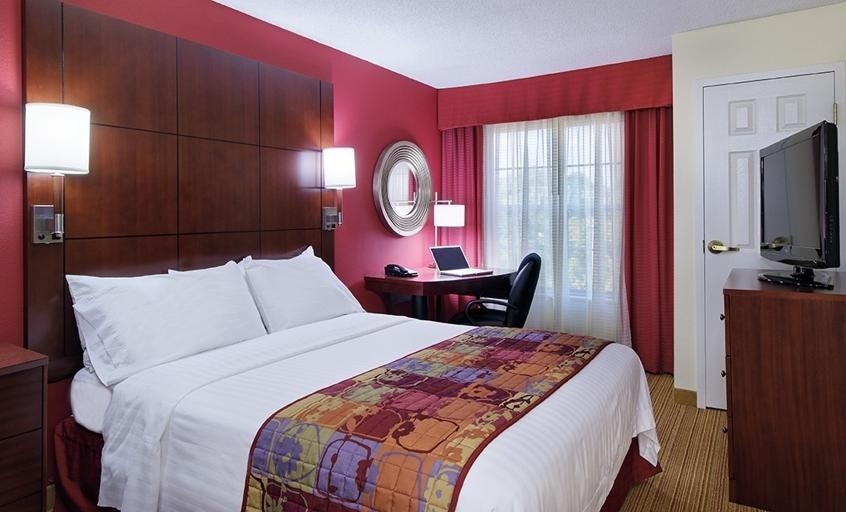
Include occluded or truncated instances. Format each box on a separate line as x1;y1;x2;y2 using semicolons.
447;253;542;328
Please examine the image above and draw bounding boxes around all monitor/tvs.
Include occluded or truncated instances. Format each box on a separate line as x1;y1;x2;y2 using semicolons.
758;119;841;291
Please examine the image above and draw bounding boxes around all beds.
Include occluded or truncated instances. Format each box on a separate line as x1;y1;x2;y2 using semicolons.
19;0;662;512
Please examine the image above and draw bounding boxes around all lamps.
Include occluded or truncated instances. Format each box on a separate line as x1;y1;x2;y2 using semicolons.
21;101;91;245
433;191;465;245
321;147;356;231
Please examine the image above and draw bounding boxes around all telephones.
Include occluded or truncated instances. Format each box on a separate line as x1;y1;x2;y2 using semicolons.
384;264;418;278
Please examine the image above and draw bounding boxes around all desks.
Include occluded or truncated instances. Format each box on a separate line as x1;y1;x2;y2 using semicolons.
365;266;516;319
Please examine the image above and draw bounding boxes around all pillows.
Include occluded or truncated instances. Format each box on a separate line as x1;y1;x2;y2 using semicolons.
242;255;368;333
66;253;267;387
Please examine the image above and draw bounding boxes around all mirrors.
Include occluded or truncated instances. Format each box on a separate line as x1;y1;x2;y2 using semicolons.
372;140;431;238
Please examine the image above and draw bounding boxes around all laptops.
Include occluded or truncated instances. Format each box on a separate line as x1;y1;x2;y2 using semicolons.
429;245;494;277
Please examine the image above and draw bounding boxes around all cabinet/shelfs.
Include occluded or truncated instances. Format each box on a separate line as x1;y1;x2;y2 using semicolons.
719;268;846;512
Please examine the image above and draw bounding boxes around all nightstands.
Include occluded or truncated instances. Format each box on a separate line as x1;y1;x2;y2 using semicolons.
0;338;49;512
